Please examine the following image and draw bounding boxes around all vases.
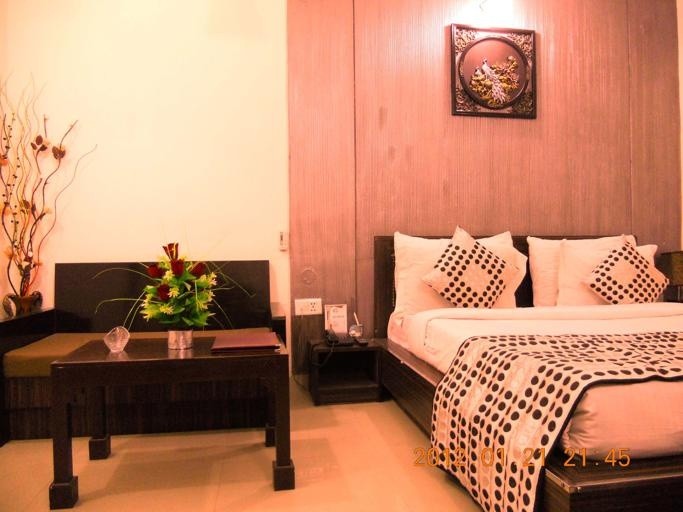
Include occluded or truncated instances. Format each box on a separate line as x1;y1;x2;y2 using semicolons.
168;329;194;350
6;291;41;317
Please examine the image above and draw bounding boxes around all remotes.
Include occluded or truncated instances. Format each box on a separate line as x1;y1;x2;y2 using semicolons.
354;334;368;346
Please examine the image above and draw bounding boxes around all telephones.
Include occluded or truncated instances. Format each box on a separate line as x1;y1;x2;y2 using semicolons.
310;328;355;367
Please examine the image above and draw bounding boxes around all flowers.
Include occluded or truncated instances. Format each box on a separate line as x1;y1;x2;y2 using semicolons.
0;69;97;295
95;242;258;330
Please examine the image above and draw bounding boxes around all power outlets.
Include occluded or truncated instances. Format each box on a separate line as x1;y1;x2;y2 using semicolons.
295;298;322;316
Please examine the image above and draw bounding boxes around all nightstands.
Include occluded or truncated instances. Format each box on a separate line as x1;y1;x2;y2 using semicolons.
308;339;384;407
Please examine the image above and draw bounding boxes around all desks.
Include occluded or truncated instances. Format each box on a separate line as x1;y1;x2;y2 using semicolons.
49;337;292;510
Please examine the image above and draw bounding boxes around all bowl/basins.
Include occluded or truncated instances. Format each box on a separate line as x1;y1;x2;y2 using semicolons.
104;326;130;353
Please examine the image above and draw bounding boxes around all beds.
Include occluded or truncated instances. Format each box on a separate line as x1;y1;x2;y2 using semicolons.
373;232;683;512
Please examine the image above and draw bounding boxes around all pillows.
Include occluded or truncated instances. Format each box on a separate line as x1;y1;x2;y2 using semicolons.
392;231;519;315
558;239;657;309
422;226;519;309
525;234;637;306
581;233;668;305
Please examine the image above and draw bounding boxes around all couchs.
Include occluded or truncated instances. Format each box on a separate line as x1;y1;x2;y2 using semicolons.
1;260;285;440
